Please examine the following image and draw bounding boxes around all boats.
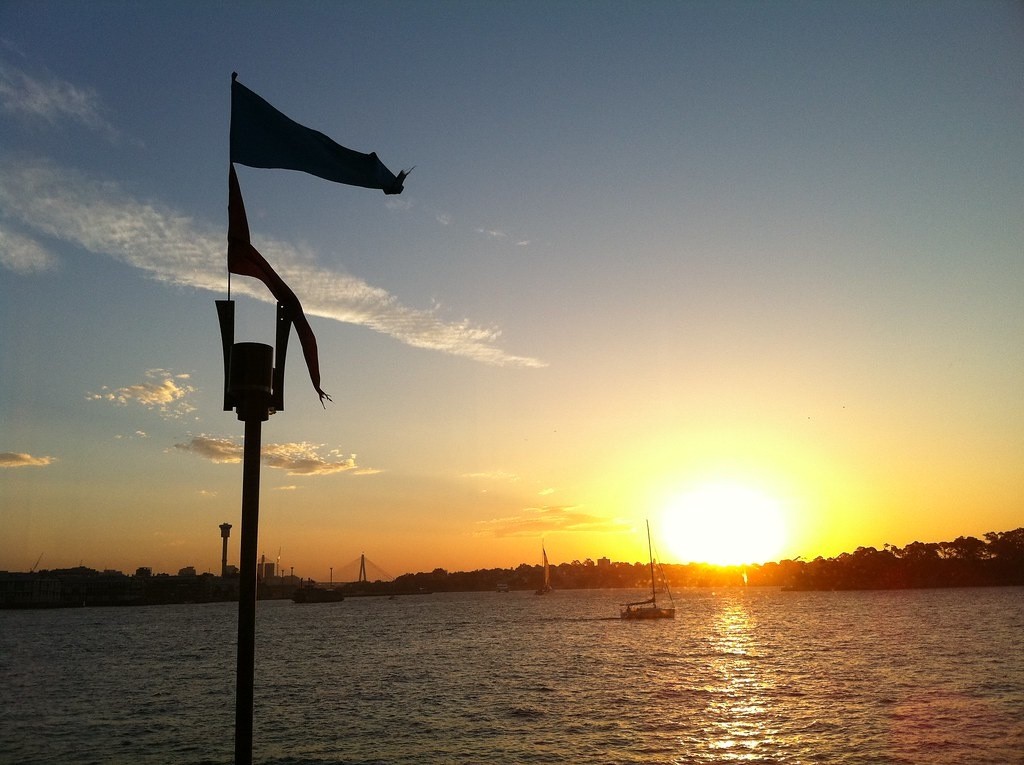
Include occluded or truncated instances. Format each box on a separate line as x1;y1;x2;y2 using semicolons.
291;584;344;602
496;583;509;592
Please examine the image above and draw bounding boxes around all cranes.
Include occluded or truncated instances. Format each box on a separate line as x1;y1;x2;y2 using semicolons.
29;553;43;572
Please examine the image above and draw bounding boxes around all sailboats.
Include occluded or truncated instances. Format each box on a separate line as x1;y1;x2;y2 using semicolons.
535;548;552;595
650;565;666;593
619;520;676;620
